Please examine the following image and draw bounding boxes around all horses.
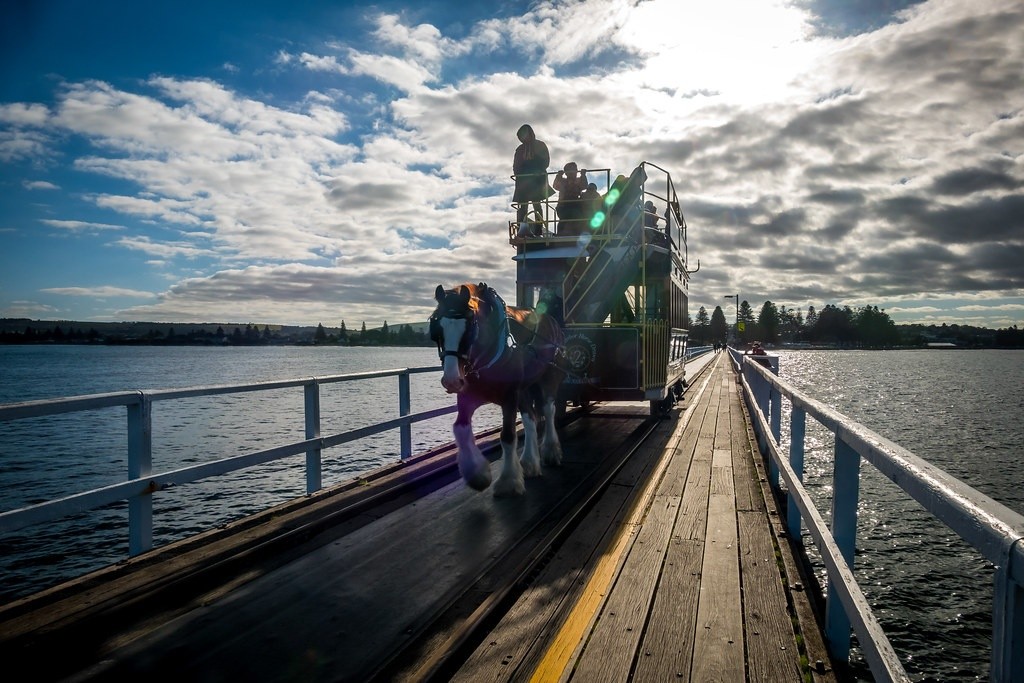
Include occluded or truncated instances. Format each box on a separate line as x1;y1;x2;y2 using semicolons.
425;282;569;499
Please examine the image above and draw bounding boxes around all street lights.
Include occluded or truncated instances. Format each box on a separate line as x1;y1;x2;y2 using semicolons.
724;294;739;350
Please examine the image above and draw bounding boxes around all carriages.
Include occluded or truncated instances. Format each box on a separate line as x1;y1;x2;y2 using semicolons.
428;161;699;497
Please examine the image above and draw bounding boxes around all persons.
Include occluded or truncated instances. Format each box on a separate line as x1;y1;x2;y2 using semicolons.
645;201;655;243
555;162;587;232
581;182;601;198
534;284;564;326
713;340;727;354
513;122;549;236
651;207;659;226
